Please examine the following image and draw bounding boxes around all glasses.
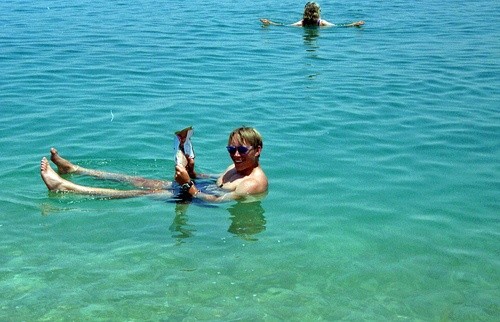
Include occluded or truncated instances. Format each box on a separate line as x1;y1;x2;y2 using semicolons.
226;145;254;154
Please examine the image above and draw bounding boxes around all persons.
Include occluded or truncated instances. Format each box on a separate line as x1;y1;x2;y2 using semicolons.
258;2;365;29
39;127;268;208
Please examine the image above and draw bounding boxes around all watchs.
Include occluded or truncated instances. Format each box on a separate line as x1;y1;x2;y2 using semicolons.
182;181;194;191
